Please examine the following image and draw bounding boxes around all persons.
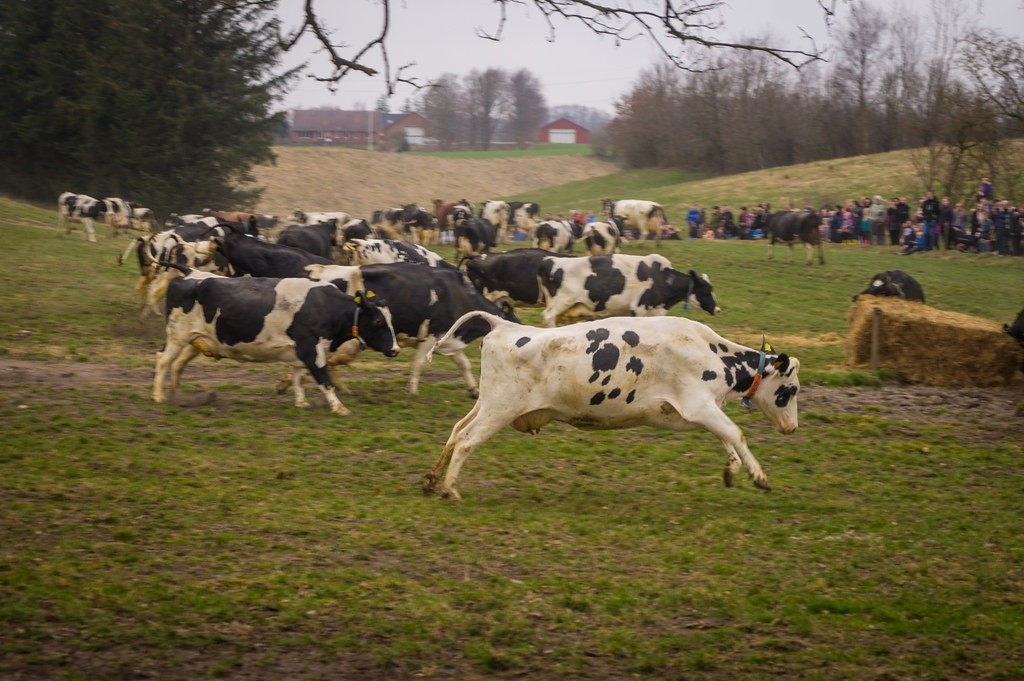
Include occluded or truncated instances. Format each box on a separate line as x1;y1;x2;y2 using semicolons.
852;269;925;304
447;197;472;248
798;204;815;266
542;176;1024;256
395;137;409;152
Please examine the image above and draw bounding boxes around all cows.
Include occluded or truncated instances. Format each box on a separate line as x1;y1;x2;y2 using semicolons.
535;252;721;329
424;308;801;503
757;210;825;267
47;191;669;321
275;260;525;400
853;270;926;304
135;235;401;416
